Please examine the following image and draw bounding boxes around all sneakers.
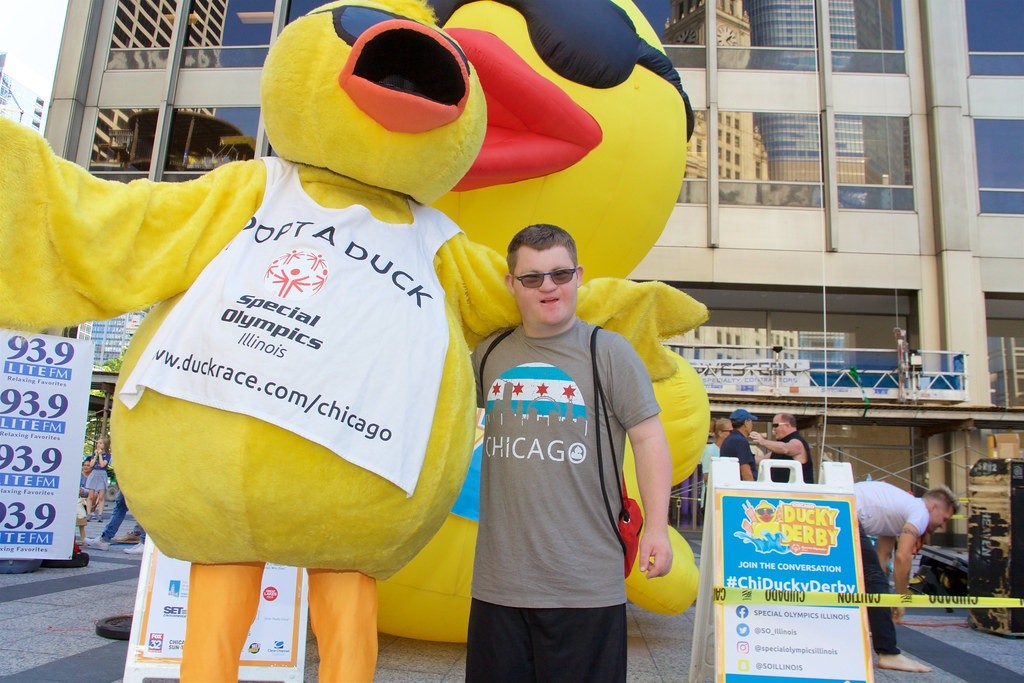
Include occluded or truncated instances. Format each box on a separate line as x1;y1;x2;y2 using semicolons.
123;543;144;554
84;538;109;551
116;532;141;543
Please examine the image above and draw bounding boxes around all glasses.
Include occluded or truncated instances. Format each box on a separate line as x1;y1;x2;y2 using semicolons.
512;268;577;288
772;423;790;428
721;430;734;434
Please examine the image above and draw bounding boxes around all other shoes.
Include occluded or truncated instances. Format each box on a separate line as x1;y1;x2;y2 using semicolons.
97;514;103;522
86;515;92;521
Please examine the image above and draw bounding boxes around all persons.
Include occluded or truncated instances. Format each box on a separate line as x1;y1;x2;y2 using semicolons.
465;223;672;683
701;418;733;507
850;481;960;672
749;414;814;484
86;437;111;522
83;488;146;554
719;409;758;481
78;456;100;520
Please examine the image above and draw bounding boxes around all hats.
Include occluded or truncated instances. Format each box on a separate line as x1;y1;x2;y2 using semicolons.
730;409;758;423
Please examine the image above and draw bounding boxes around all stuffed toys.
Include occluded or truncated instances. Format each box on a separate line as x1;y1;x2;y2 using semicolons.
375;1;710;644
0;0;708;683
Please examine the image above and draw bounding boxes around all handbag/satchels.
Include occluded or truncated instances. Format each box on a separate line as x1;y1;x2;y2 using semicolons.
618;497;643;579
